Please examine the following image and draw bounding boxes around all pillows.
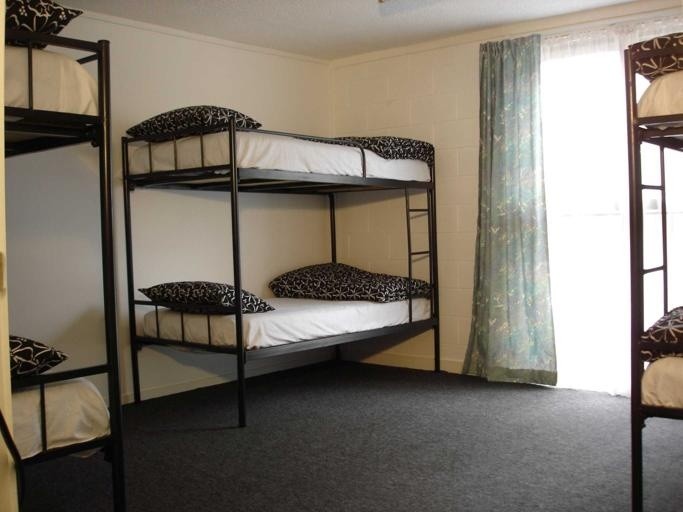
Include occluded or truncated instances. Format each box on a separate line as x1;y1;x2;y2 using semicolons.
4;0;84;49
125;103;260;142
134;281;273;316
6;332;70;395
625;31;681;81
639;304;682;362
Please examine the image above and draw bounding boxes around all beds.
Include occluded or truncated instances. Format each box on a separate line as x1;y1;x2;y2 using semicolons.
1;24;127;511
620;40;682;511
120;116;443;427
